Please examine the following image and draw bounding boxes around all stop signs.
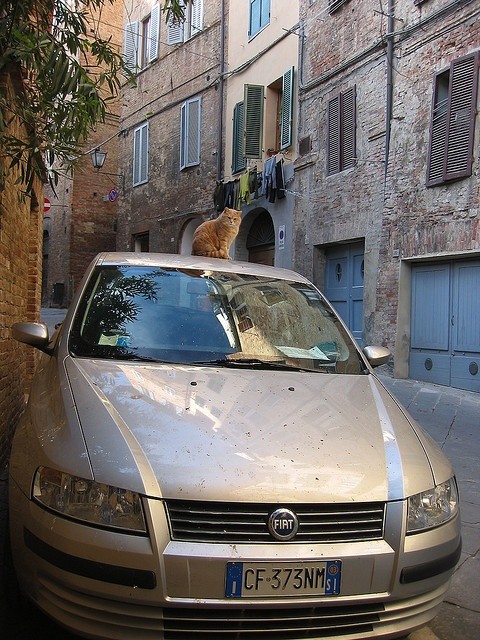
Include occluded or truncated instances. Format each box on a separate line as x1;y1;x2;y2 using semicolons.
44;198;51;212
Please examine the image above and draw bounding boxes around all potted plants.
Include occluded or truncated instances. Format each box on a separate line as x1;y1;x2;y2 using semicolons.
266;148;275;156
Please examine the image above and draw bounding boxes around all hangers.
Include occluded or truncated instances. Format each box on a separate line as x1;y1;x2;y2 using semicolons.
281;152;292;164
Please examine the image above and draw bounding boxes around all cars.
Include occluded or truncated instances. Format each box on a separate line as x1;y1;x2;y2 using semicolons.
9;251;462;640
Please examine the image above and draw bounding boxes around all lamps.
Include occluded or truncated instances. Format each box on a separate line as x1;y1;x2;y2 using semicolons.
91;145;126;194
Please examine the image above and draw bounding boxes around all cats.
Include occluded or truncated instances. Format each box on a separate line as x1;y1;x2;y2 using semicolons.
191;207;244;261
